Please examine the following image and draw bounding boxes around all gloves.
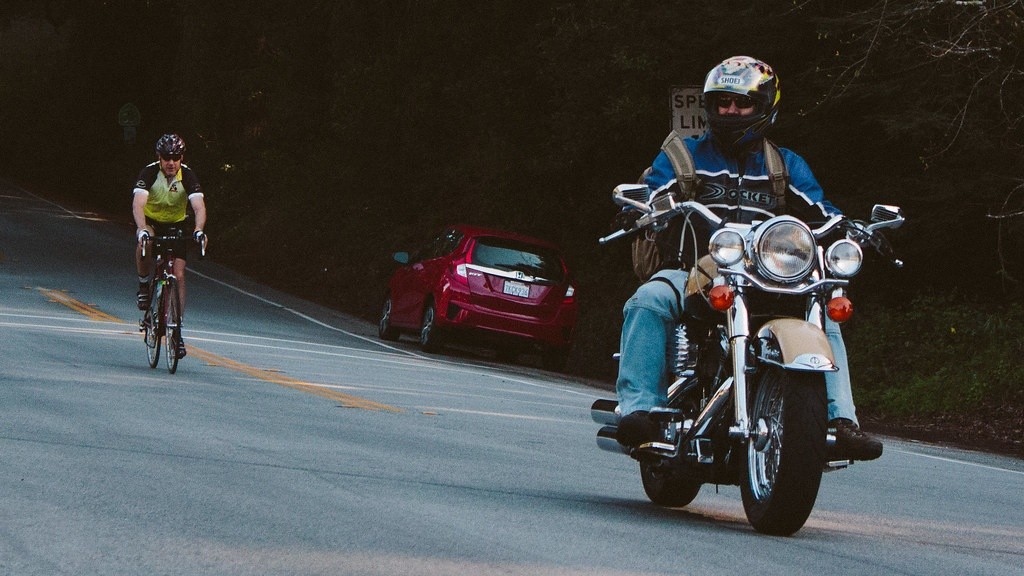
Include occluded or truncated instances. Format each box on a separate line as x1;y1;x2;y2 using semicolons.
609;209;642;232
843;219;891;253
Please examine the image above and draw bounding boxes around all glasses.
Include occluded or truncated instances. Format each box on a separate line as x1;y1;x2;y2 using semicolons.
717;93;756;109
160;154;182;162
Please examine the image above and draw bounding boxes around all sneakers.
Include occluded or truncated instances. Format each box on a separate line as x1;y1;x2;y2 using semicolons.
617;412;661;446
137;282;150;310
171;335;186;356
829;418;883;461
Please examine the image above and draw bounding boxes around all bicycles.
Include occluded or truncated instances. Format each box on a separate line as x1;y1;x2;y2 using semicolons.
136;229;206;375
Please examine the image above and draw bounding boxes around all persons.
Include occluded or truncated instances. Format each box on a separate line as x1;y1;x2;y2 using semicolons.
617;51;883;462
131;133;208;358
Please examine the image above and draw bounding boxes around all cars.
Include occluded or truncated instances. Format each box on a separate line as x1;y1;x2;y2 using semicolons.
376;222;579;365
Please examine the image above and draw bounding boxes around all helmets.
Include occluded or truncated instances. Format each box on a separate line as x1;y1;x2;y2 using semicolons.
702;55;780;151
156;133;186;155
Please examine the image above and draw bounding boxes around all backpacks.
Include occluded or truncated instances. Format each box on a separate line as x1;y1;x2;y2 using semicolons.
632;130;789;283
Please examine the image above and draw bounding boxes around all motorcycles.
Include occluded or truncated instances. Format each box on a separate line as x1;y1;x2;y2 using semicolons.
589;180;906;536
